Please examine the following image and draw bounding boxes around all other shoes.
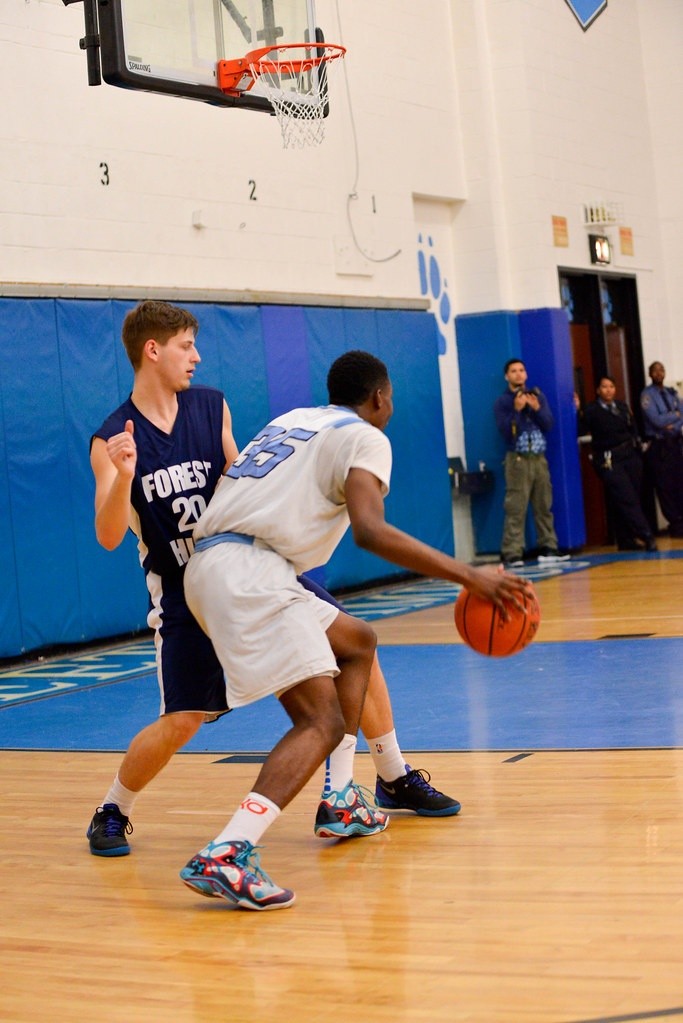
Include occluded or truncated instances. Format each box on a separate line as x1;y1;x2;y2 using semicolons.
508;557;525;567
644;534;658;552
617;540;645;551
537;546;571;561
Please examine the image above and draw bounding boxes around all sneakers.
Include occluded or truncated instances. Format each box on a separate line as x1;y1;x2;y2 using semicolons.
86;801;134;855
178;839;296;911
314;783;389;837
374;764;461;817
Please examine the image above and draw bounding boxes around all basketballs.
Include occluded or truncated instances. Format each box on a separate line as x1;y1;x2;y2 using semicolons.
455;573;542;659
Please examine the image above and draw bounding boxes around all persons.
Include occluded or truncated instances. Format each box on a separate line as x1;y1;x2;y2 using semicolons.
180;352;534;911
86;301;460;857
574;361;683;551
495;359;570;567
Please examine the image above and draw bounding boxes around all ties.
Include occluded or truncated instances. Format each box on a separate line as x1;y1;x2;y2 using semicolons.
659;390;671;412
606;403;613;413
